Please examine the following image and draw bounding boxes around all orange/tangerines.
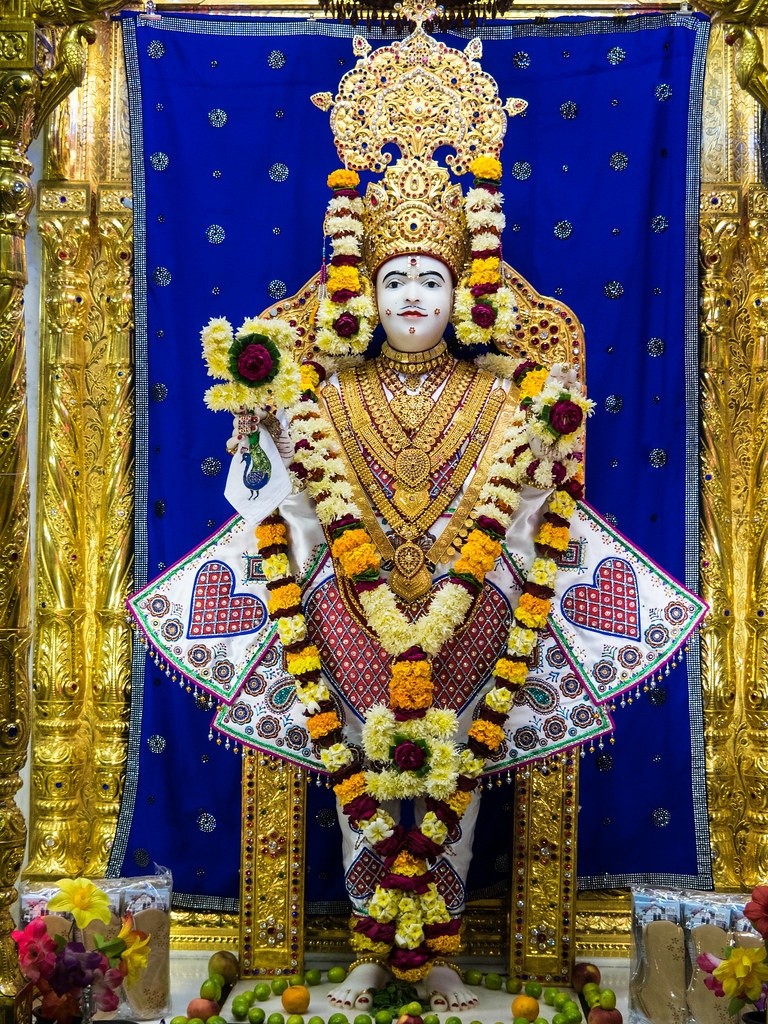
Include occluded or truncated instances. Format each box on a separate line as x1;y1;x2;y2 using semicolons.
511;994;540;1022
282;985;309;1013
169;966;617;1024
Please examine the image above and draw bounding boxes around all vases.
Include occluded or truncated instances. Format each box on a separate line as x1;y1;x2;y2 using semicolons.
741;1010;768;1024
32;1004;84;1024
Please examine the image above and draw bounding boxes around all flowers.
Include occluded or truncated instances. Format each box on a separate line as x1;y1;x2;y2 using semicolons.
697;884;768;1024
202;152;599;984
12;874;154;1018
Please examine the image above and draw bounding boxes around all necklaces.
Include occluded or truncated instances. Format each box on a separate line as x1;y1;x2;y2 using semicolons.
319;337;508;607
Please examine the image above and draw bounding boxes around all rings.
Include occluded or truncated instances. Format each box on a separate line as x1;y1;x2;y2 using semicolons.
359;988;377;997
428;990;448;1000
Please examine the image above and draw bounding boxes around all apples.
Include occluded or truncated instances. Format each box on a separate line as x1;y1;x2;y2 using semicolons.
571;963;601;991
588;1005;623;1024
186;997;219;1021
397;1013;423;1024
208;951;238;983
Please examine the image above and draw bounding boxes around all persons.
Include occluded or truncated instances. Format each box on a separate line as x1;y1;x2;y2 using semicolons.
127;1;709;1013
